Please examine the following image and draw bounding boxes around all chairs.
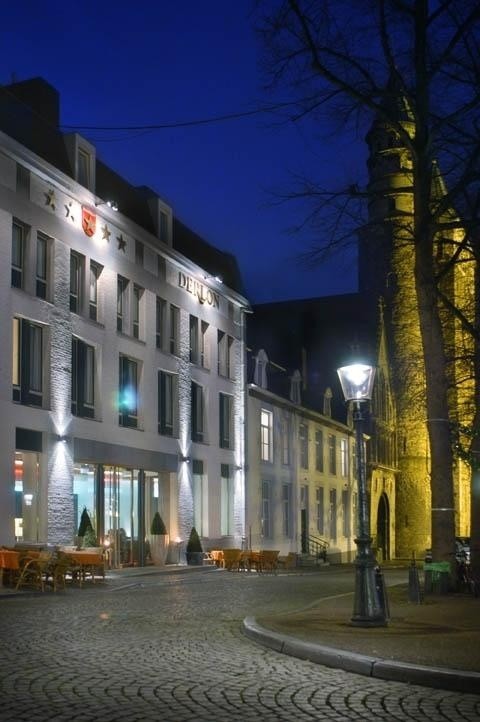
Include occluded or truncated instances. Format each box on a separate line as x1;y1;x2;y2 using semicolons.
15;545;106;593
202;549;297;576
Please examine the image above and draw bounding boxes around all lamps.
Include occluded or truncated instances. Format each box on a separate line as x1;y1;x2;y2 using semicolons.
205;275;223;283
95;200;119;212
15;518;23;542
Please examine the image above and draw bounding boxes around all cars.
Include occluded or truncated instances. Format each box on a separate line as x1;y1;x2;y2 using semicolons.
426;537;471;567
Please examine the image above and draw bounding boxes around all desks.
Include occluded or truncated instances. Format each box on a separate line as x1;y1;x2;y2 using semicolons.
0;549;40;588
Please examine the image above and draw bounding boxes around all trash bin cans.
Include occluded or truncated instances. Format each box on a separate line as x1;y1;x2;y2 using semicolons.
423;563;450;595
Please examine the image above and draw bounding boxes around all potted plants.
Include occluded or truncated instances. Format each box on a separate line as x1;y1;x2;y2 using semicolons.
149;512;169;566
185;527;204;566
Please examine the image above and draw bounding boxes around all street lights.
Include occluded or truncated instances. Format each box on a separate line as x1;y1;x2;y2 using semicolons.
335;358;391;628
